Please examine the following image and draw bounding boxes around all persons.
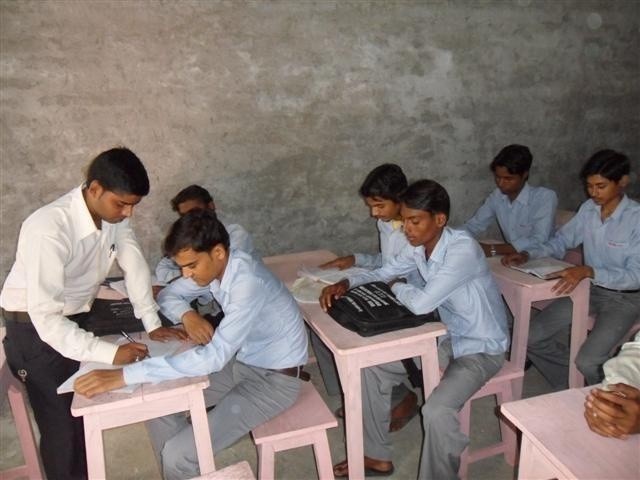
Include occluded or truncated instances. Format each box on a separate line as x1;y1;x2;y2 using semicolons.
581;327;640;442
72;207;314;480
459;142;555;257
318;176;512;480
499;148;640;391
152;183;264;284
316;161;424;290
0;147;191;479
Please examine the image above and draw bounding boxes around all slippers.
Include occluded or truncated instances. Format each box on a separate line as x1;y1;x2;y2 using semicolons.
334;460;394;479
388;405;422;434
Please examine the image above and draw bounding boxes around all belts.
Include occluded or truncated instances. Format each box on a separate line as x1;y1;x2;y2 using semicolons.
267;365;311;382
594;284;638;294
1;307;35;324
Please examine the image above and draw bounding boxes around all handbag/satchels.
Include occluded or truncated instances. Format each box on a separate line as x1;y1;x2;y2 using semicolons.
326;280;435;339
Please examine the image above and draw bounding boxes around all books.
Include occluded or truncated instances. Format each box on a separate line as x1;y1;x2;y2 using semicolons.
108;276;167;297
509;255;576;281
54;360;140;396
296;263;369;286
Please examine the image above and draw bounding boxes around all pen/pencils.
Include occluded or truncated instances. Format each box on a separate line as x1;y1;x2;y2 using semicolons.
120;328;152;359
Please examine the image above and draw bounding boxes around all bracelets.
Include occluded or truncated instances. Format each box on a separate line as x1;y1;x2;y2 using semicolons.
490;245;497;256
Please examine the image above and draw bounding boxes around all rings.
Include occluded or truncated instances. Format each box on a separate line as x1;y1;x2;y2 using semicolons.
135;357;139;362
593;412;597;418
